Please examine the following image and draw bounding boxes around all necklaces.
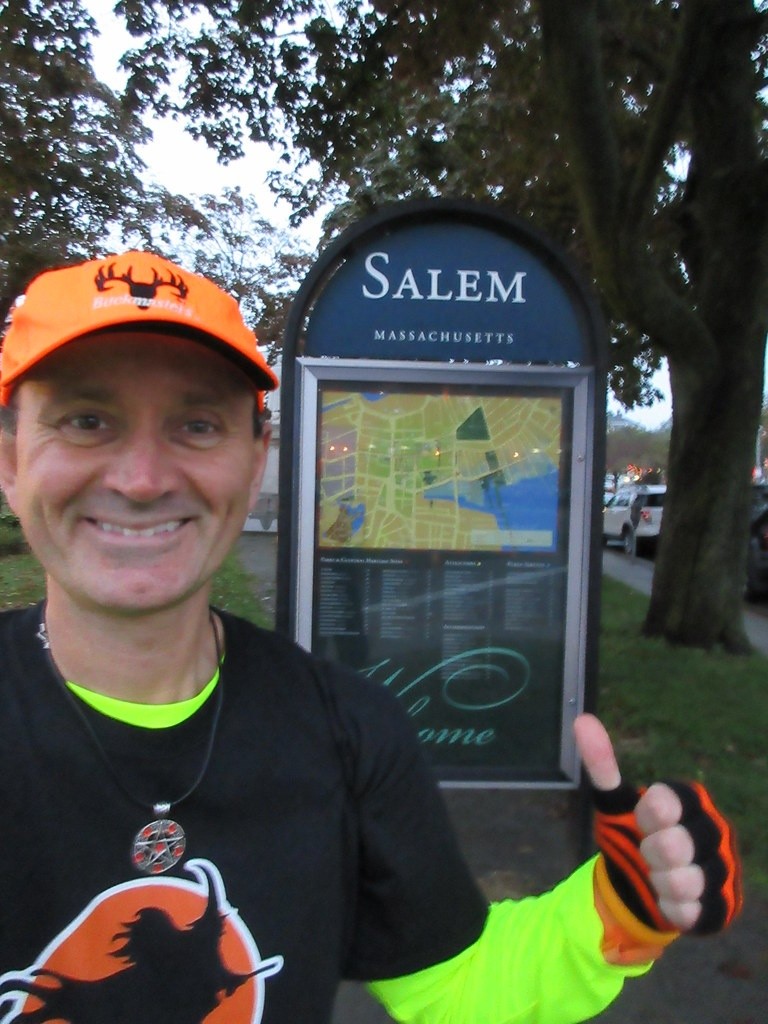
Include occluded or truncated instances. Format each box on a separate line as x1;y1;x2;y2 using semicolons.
38;602;223;874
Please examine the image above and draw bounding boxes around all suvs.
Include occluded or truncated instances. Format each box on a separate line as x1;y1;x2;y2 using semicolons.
602;485;668;556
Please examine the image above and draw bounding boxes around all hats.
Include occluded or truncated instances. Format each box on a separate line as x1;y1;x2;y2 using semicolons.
0;252;280;414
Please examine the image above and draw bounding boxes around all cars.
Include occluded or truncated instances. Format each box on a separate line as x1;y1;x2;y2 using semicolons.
744;485;768;604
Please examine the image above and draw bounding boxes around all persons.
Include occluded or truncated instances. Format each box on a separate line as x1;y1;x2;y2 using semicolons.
0;250;743;1024
